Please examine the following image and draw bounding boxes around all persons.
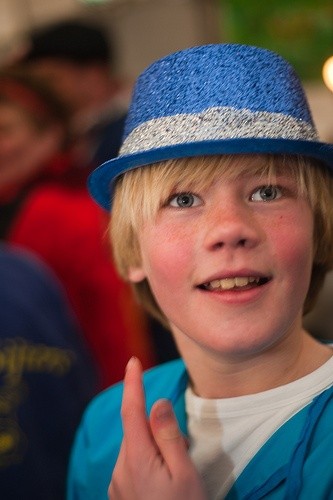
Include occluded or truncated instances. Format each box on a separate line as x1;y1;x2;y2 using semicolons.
0;15;154;500
66;43;333;500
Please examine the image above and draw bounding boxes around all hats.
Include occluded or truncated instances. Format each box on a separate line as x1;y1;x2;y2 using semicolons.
86;40;333;212
